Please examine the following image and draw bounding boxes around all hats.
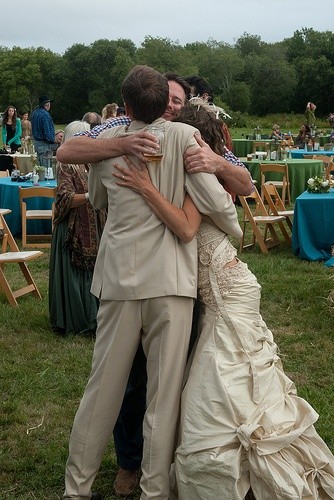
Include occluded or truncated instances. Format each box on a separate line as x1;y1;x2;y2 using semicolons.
36;96;54;106
117;107;126;115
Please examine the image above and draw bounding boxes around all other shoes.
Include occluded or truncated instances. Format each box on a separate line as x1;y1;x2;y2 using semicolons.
84;329;96;338
52;325;66;335
113;467;138;495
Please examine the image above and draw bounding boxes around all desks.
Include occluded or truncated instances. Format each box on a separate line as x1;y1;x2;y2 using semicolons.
0;176;57;240
231;138;274;157
236;157;324;203
0;153;33;174
290;187;334;267
287;149;334;159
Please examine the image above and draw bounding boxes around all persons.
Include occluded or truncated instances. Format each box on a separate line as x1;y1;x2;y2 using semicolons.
268;124;286;141
61;64;244;500
20;112;32;140
111;95;334;500
324;156;334;180
30;95;64;167
293;124;314;149
305;102;316;129
48;73;256;500
1;106;22;153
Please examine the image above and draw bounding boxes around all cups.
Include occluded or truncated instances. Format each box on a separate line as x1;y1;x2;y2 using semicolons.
33;175;40;186
247;153;252;161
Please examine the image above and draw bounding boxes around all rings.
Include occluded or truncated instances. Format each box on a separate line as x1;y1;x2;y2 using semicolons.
123;176;124;179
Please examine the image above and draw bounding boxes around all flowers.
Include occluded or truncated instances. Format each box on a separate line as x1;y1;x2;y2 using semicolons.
307;176;331;193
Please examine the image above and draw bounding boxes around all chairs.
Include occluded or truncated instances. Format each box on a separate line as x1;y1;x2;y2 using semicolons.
0;212;44;308
19;186;56;248
237;141;333;256
0;208;12;239
